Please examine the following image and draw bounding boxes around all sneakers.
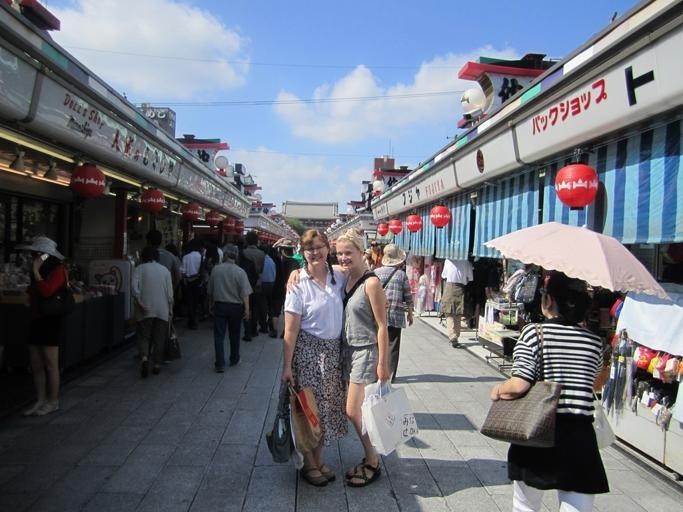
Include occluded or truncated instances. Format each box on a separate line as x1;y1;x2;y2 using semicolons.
141;357;148;378
23;399;59;416
300;462;336;487
153;364;160;374
242;326;278;341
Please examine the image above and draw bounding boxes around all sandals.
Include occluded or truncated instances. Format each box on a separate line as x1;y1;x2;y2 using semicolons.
345;458;380;487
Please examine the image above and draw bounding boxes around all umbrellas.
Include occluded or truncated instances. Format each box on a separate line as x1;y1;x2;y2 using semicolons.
482;220;672;304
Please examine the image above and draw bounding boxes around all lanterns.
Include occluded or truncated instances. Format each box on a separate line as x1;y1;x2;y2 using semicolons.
388;219;402;234
207;211;219;225
235;223;244;232
142;188;164;214
429;206;450;227
555;164;597;210
224;217;235;228
406;215;421;232
72;163;106;199
181;202;201;221
378;224;388;236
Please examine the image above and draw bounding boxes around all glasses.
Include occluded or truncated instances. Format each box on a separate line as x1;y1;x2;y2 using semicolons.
304;244;325;252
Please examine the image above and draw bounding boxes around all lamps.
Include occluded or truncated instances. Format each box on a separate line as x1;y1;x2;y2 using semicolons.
9;149;25;171
43;159;57;180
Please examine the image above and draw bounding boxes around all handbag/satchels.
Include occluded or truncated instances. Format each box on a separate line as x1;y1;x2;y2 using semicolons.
163;334;181;360
266;410;294;463
503;270;539;303
39;287;74;315
361;387;418;456
635;344;683;383
592;405;615;448
291;388;323;449
481;380;561;448
183;276;206;296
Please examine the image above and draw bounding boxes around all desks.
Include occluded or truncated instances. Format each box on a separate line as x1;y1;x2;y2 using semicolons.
0;291;125;417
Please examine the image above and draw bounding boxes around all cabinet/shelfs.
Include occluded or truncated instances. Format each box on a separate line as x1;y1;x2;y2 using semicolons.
478;298;520;358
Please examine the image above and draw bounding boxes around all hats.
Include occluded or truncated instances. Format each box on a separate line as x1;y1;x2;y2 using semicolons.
14;237;64;260
276;239;296;249
382;244;407;266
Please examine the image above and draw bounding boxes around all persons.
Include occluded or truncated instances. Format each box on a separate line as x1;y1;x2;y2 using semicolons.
491;271;609;512
14;236;69;415
130;227;475;377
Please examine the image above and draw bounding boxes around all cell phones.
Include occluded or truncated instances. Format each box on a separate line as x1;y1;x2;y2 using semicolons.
39;253;50;262
